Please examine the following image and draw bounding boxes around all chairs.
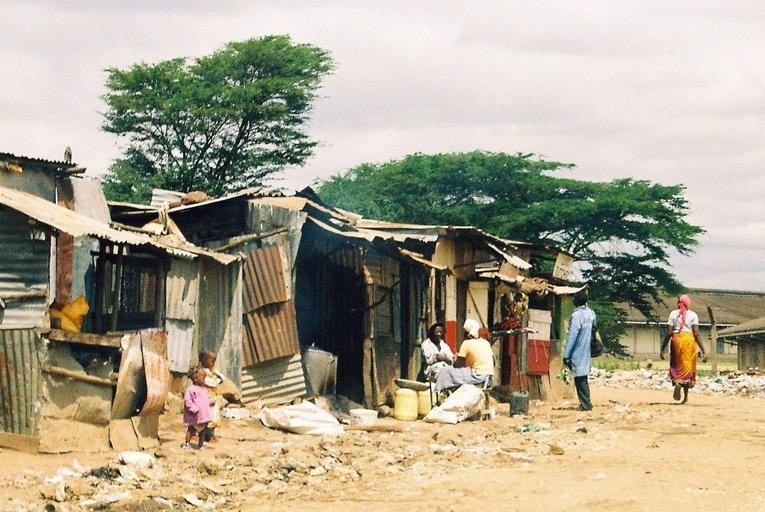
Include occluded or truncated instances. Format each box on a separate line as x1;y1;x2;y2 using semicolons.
440;375;494;423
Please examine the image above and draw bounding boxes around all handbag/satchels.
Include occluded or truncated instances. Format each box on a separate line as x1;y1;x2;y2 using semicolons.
591;340;602;358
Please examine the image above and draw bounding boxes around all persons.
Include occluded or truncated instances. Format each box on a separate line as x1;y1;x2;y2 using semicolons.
435;318;496;422
200;348;224;444
418;323;454;380
660;293;709;403
562;290;598;410
182;365;216;453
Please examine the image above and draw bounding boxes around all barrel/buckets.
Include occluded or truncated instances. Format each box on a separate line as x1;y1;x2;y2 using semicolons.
510;390;529;416
